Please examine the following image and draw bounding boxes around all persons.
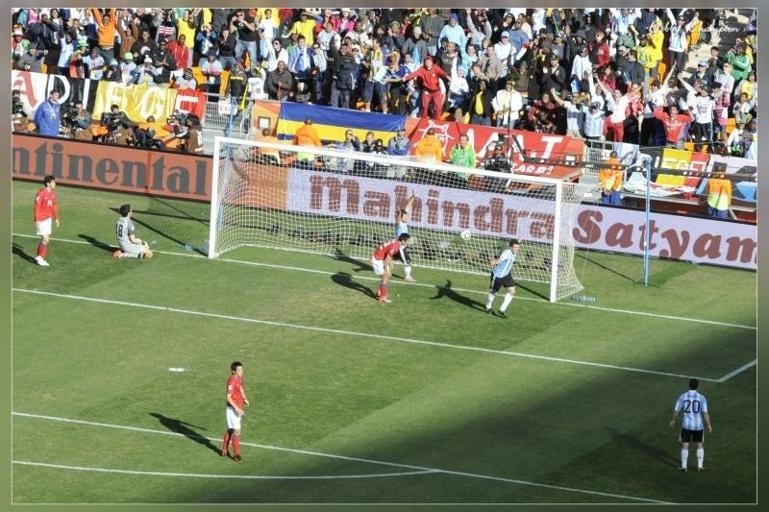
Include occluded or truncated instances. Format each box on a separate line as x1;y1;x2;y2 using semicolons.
111;203;153;260
14;6;758;222
33;174;61;268
367;233;413;304
221;361;250;462
389;189;418;283
482;235;525;318
669;379;712;473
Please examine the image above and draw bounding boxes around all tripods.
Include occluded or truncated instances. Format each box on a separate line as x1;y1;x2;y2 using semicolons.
62;124;73;138
105;130;117;144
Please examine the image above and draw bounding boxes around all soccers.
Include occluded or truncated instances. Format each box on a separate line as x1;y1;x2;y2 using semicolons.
461;230;472;240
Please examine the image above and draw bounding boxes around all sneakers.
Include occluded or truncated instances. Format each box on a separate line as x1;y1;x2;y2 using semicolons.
223;455;232;461
113;250;124;258
376;296;392;303
234;457;243;463
696;467;706;472
484;304;508;318
34;256;50;267
677;465;687;472
404;276;416;282
